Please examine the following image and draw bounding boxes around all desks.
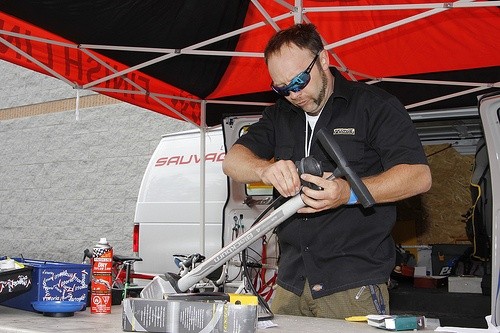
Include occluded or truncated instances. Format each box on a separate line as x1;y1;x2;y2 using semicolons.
255;314;500;333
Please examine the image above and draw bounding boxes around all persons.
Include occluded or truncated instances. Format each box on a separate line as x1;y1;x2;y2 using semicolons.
222;24;433;319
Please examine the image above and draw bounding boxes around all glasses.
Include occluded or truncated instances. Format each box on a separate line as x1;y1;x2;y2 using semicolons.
271;49;321;96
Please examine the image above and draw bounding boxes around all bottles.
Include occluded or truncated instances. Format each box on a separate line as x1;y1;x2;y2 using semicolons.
90;238;113;314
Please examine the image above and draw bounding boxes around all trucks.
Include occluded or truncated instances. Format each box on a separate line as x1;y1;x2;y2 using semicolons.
127;91;500;333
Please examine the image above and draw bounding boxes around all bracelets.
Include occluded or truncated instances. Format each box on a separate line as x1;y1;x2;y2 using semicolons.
347;188;358;205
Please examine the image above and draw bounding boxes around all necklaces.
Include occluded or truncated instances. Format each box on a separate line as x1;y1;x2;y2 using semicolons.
304;92;333;157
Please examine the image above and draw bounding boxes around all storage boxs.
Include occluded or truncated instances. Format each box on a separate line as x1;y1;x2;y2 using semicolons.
0;254;93;314
